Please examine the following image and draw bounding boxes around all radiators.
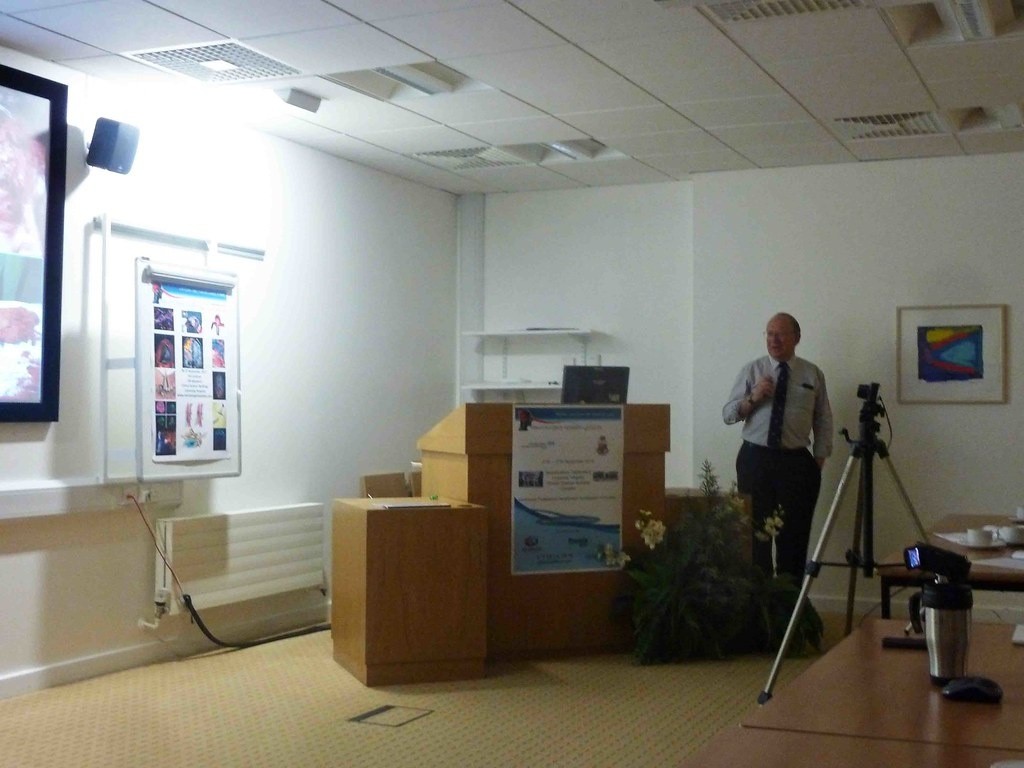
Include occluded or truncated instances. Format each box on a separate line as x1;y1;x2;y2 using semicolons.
138;502;325;632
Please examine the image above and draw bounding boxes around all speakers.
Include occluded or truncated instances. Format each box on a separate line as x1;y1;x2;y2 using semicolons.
86;117;139;176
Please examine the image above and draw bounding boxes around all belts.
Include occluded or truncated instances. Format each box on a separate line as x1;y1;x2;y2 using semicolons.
743;440;766;450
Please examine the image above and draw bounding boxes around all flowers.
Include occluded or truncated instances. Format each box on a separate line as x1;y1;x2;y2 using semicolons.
593;458;826;666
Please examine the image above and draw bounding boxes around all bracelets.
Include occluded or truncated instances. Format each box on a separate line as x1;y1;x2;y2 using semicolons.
747;394;755;405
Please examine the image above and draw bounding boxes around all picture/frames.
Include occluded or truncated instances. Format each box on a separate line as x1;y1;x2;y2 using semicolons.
0;64;69;421
894;303;1007;406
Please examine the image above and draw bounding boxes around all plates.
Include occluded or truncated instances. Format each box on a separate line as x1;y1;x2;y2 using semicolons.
1008;518;1024;522
957;538;1006;550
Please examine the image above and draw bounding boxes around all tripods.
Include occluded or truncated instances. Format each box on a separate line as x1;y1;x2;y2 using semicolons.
756;404;931;708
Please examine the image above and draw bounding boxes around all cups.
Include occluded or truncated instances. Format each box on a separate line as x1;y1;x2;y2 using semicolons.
1017;506;1024;519
967;528;992;545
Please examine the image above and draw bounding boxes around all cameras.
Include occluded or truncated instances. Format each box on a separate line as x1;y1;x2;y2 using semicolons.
904;539;972;584
856;382;881;404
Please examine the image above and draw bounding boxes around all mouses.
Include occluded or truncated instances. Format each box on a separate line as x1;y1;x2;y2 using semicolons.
940;676;1003;703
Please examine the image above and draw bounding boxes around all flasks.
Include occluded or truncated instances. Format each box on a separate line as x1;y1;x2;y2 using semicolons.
921;583;974;685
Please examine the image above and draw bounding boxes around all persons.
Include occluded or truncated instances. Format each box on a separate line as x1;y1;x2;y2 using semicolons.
723;313;832;590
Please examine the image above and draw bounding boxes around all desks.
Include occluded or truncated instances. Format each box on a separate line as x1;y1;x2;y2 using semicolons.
676;611;1024;768
331;497;485;688
878;513;1024;619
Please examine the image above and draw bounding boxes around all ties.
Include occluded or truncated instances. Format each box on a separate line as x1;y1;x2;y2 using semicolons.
767;361;789;451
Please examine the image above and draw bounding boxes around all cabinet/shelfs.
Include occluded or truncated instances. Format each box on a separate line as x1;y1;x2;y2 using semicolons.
459;328;591;402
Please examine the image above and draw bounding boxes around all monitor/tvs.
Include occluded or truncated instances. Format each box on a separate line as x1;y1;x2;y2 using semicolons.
560;366;630;405
